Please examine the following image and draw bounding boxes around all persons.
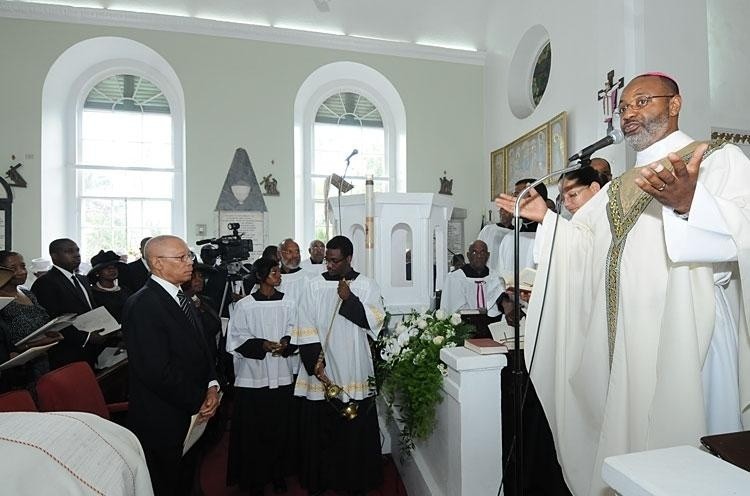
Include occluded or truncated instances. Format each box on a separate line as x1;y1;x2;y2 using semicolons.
225;258;299;496
0;238;327;408
290;236;387;496
122;235;221;496
495;75;750;496
440;157;612;339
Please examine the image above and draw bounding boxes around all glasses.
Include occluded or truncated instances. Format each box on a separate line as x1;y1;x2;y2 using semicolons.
613;96;674;118
158;255;196;262
324;256;345;265
557;185;589;206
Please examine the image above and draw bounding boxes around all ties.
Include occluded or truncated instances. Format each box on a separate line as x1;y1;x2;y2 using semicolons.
71;276;89;307
177;290;195;328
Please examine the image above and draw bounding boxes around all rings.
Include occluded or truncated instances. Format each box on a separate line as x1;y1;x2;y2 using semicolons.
659;183;666;191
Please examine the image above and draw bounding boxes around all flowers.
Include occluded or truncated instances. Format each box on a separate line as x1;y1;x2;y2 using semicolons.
367;308;478;465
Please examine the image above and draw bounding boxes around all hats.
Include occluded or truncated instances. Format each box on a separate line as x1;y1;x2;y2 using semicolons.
87;250;128;283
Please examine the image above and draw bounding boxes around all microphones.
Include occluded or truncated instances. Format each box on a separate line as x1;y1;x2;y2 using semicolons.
567;126;627;163
345;149;359;162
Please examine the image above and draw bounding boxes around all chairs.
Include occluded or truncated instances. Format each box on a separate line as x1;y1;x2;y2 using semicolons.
36;361;129;420
0;388;37;412
